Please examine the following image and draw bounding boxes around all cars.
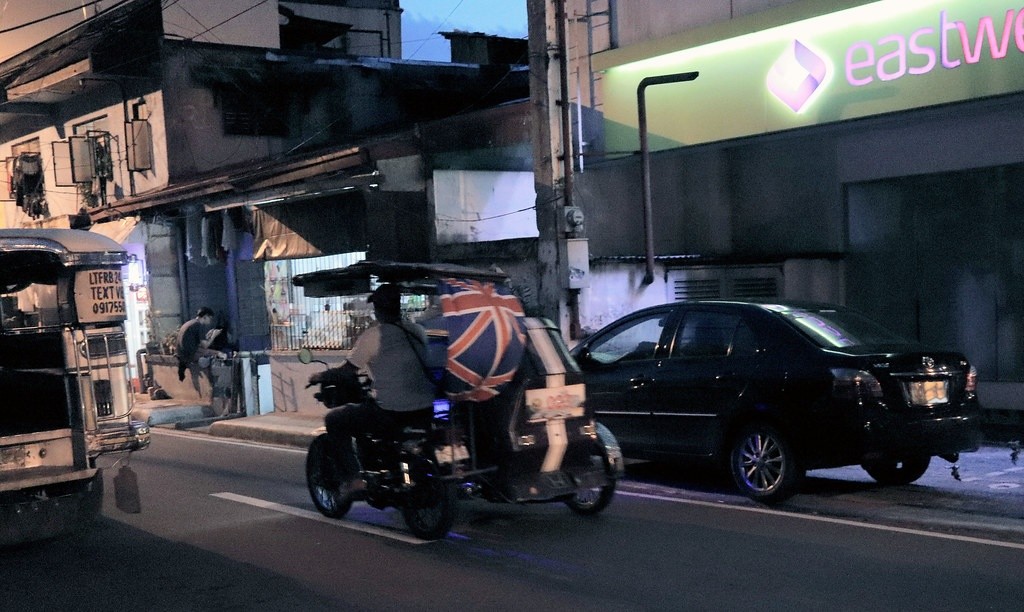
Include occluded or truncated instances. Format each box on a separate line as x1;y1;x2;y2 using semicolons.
563;303;977;504
4;228;151;493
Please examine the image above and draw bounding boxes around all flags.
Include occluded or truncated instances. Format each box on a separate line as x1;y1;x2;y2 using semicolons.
436;277;527;403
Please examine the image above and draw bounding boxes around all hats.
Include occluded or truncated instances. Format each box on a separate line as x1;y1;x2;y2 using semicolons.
367;284;401;303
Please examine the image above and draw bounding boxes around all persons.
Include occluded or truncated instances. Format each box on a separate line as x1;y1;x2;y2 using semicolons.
308;285;436;496
176;307;227;362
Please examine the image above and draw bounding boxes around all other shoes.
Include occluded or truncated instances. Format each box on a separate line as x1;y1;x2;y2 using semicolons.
339;480;368;493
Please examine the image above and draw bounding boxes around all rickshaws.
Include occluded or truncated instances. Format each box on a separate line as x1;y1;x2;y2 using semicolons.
297;261;586;536
